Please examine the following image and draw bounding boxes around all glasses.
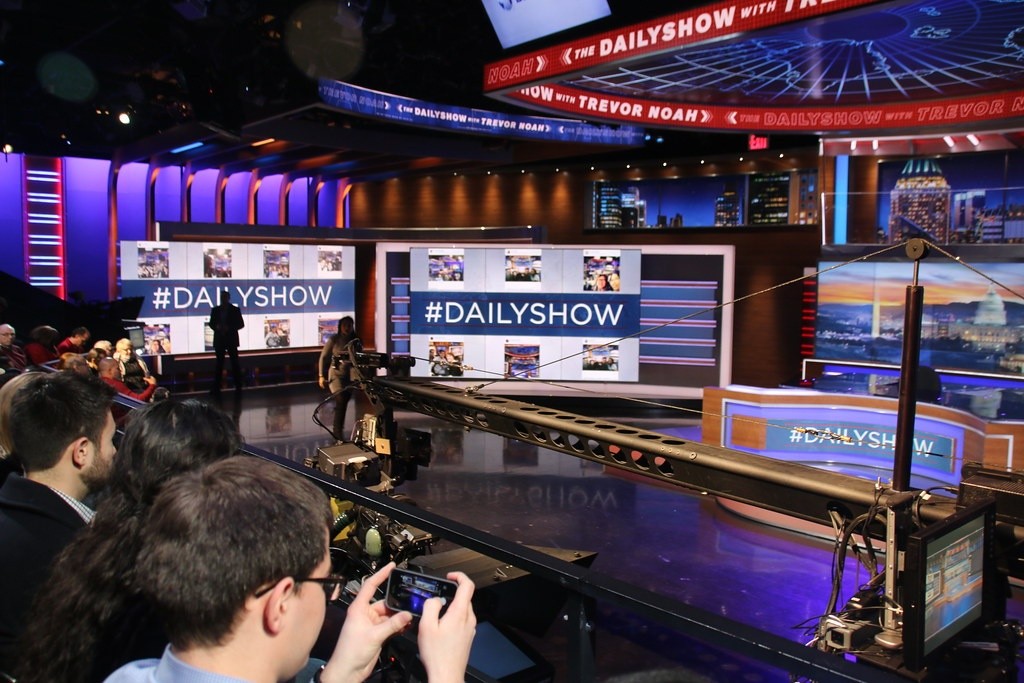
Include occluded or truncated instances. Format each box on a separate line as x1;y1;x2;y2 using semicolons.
255;573;347;602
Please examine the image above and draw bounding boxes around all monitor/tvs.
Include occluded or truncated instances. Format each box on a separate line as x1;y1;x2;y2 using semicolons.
482;0;612;50
902;497;997;673
126;326;145;349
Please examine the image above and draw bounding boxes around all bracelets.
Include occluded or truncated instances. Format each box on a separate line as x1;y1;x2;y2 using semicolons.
314;664;325;683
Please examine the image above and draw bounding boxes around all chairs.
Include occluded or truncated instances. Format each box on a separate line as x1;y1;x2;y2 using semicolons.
913;367;945;402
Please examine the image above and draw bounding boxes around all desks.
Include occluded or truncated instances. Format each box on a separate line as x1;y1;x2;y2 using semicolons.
702;387;1024;485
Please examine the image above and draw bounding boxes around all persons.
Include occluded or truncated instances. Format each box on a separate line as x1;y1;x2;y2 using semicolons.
0;324;169;454
209;291;244;388
437;269;464;281
264;263;289;278
583;357;616;369
318;257;343;271
593;263;619;290
319;316;364;443
430;349;464;376
505;268;540;281
0;370;116;634
105;456;475;683
14;399;238;683
209;265;231;278
264;324;286;341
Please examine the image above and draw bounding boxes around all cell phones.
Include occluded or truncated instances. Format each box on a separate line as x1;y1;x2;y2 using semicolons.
386;567;459;619
